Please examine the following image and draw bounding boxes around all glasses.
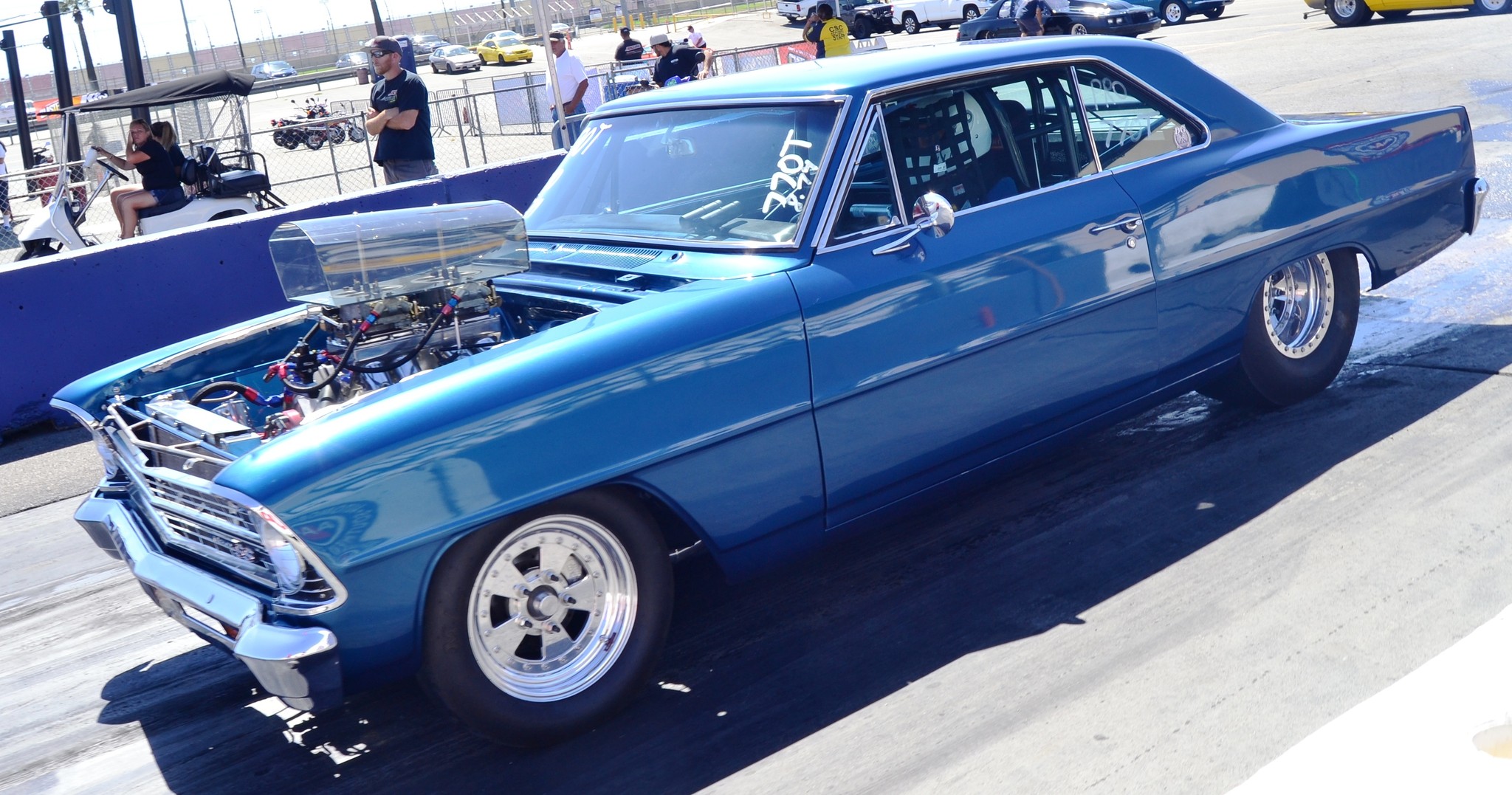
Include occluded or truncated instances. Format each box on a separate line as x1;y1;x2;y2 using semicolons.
370;50;394;58
650;45;654;49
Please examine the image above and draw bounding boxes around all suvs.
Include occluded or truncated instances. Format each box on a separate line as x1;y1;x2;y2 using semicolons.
410;35;451;62
816;0;892;40
251;61;298;81
0;100;36;124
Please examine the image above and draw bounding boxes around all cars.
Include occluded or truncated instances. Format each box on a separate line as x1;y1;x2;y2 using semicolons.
534;23;570;46
1305;0;1512;27
892;0;1236;39
428;45;481;75
336;51;372;74
49;36;1490;751
477;29;533;67
615;42;679;74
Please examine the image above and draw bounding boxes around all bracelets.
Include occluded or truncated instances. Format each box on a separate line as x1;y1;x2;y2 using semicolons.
1040;25;1044;27
807;19;812;23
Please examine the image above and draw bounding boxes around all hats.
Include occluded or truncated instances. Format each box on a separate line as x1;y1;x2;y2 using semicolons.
650;33;669;46
687;25;692;30
549;29;564;41
365;35;403;56
621;28;631;33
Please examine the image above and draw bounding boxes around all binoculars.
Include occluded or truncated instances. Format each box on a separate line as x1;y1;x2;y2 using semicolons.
677;199;742;234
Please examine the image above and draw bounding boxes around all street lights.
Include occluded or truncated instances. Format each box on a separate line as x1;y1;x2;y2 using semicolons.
253;9;267;54
187;20;198;50
320;0;333;24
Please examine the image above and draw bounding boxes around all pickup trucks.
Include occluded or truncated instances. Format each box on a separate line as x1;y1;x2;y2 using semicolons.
776;0;817;24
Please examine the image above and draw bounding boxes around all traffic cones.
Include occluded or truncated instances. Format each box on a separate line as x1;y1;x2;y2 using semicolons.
463;107;468;124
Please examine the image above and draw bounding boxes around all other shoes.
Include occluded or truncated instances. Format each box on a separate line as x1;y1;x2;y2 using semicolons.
3;224;12;232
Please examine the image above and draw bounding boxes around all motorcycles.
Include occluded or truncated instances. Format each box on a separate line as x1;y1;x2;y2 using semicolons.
269;98;365;150
639;52;705;125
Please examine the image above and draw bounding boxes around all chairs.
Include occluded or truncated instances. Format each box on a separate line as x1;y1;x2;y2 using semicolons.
985;101;1040;195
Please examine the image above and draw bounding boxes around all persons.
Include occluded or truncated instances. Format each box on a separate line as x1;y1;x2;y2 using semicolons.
687;25;706;48
829;90;993;251
649;33;714;90
116;118;185;240
802;4;852;59
1015;0;1045;37
110;121;191;242
614;27;644;66
360;35;440;185
0;141;11;230
542;30;590;149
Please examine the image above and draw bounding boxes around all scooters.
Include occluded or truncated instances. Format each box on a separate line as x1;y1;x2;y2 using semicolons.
33;142;54;167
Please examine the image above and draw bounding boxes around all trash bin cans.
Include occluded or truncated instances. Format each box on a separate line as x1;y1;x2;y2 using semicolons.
357;68;369;85
33;163;59;206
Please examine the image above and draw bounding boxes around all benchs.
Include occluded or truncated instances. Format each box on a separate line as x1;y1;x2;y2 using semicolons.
138;145;270;217
